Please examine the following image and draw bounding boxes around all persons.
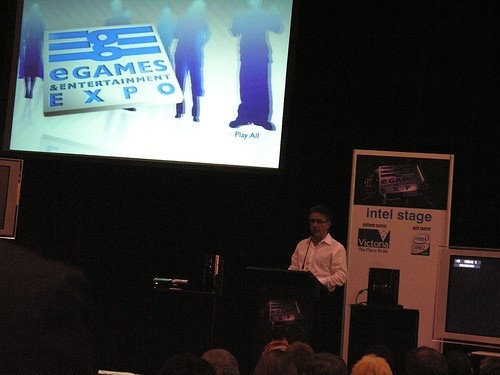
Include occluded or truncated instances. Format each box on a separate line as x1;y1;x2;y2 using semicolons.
480;356;500;375
18;0;49;99
402;346;449;375
103;0;141;112
229;0;284;132
157;6;174;70
349;354;393;375
352;343;394;370
444;349;474;375
252;338;316;375
200;347;240;375
175;0;211;122
287;204;347;293
303;351;346;375
158;351;217;375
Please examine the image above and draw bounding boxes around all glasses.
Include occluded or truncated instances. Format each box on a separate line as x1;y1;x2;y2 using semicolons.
309;218;329;225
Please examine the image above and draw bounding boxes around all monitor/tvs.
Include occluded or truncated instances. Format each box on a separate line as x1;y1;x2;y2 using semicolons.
431;244;500;357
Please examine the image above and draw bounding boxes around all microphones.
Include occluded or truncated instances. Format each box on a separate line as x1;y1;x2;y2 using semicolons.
299;231;315;271
356;283;393;304
